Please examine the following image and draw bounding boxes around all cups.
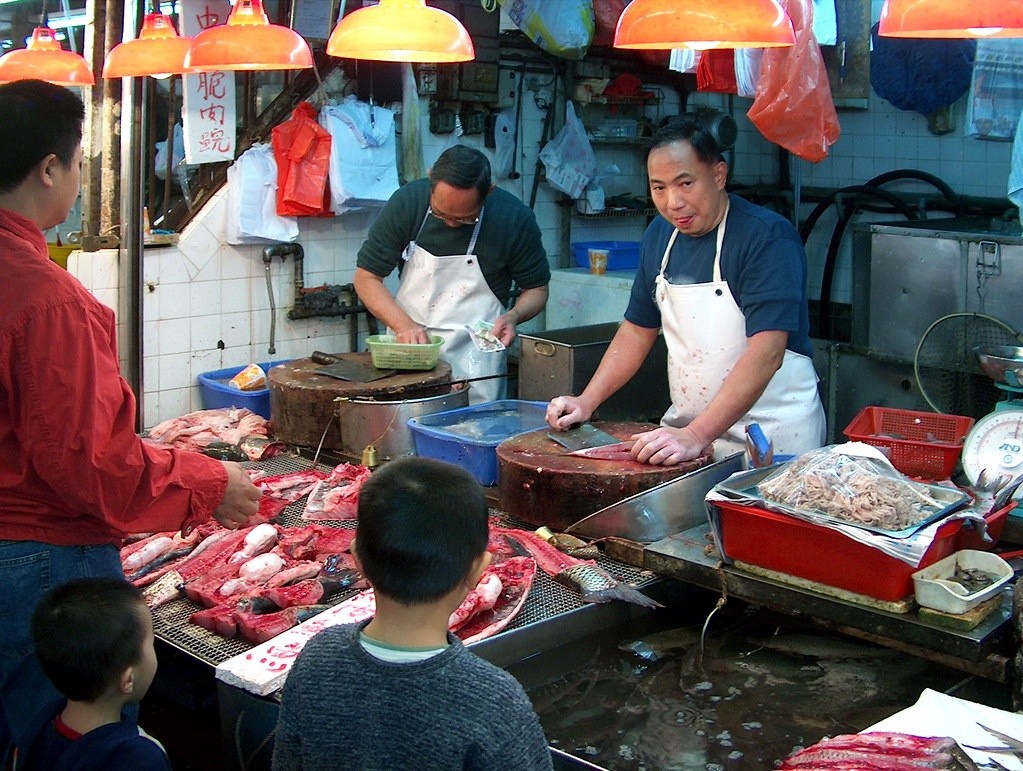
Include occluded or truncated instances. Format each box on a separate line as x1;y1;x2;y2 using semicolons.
229;364;266;391
588;248;610;274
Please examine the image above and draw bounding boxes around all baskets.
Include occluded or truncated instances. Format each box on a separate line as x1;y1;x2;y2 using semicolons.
362;333;446;371
840;405;977;481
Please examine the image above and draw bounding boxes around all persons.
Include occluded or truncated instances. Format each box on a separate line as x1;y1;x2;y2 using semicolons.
271;457;552;771
0;576;171;771
0;77;264;739
353;144;548;407
545;121;826;468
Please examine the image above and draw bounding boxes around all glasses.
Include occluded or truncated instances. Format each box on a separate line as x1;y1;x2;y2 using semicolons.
426;205;480;226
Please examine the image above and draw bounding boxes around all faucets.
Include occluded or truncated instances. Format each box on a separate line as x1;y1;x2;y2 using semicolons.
262;243;305;355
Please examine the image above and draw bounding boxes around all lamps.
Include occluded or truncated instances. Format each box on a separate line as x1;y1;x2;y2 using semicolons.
327;0;475;63
877;0;1023;38
184;0;314;70
102;0;216;80
614;0;796;50
0;0;96;87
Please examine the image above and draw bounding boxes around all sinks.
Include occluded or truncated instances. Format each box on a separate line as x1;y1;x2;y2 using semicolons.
197;359;299;421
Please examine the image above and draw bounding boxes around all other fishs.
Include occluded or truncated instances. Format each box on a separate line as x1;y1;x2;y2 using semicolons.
774;722;1023;771
117;461;668;645
136;404;287;463
558;438;639;462
928;468;1023;544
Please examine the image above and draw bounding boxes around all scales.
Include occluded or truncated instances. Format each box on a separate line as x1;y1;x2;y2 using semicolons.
952;345;1023;518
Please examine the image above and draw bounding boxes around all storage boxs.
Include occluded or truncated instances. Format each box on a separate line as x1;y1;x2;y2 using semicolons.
198;358;289;419
957;495;1018;551
572;240;647;270
708;475;967;601
408;399;552;486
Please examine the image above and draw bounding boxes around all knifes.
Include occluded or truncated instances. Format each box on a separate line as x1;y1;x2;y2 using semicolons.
547;412;621;453
311;350;395;383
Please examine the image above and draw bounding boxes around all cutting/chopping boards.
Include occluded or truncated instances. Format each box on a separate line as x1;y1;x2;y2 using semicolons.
268;352;452;449
494;420;714;530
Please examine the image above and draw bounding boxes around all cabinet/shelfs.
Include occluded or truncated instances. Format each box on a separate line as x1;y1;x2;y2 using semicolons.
562;90;663;219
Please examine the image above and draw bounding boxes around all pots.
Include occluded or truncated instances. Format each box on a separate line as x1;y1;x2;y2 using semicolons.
698;107;738;154
